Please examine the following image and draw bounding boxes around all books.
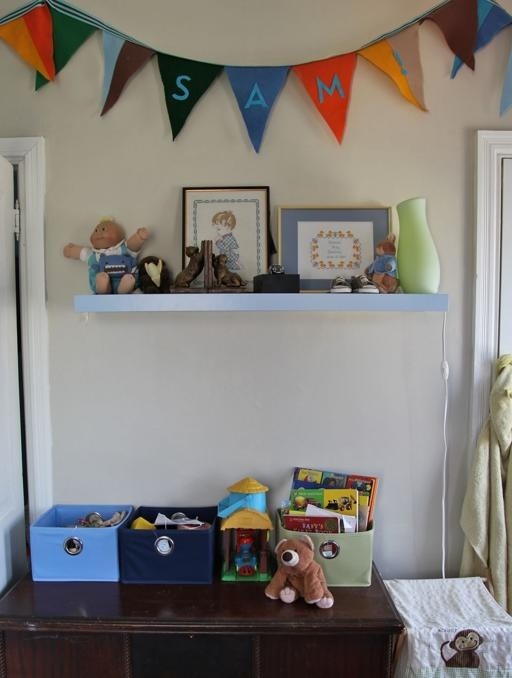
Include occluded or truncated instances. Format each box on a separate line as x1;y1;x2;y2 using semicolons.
281;466;378;557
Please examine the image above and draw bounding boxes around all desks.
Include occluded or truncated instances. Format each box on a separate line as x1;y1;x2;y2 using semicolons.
1;561;404;677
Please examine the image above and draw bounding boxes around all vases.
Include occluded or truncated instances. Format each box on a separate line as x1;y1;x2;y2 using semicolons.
395;196;441;294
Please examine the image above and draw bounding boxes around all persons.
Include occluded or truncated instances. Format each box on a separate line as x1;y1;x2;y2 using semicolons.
64;215;150;294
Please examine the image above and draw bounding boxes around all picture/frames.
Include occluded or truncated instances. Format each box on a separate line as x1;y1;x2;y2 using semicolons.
277;206;394;294
183;186;270;289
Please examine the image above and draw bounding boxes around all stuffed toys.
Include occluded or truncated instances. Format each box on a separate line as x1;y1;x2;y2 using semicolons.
265;535;335;610
365;232;399;293
